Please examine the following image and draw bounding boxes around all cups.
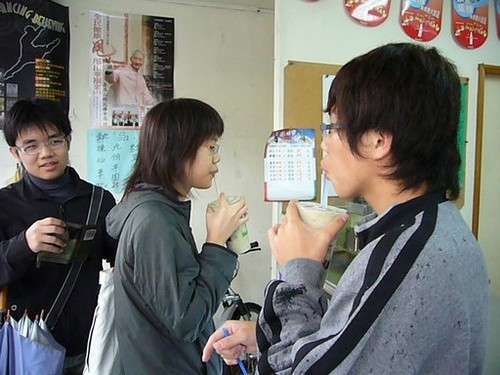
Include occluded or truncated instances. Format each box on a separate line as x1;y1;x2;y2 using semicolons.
295;201;348;272
209;194;251;255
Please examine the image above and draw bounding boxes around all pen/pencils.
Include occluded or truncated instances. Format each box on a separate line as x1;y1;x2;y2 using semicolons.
222;326;248;375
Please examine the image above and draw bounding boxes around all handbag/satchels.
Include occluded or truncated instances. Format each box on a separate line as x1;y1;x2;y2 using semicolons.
82;259;123;374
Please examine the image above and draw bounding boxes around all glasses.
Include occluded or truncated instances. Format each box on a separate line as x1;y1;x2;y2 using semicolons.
202;142;220;154
320;123;343;137
14;136;67;155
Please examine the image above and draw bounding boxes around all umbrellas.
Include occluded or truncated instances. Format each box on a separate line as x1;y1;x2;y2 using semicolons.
0;284;66;375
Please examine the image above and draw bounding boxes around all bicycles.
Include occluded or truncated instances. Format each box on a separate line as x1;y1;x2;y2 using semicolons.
220;240;262;375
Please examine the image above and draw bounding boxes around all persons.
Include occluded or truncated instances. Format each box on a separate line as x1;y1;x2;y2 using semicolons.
202;42;492;375
106;97;251;375
0;95;118;375
104;49;159;105
